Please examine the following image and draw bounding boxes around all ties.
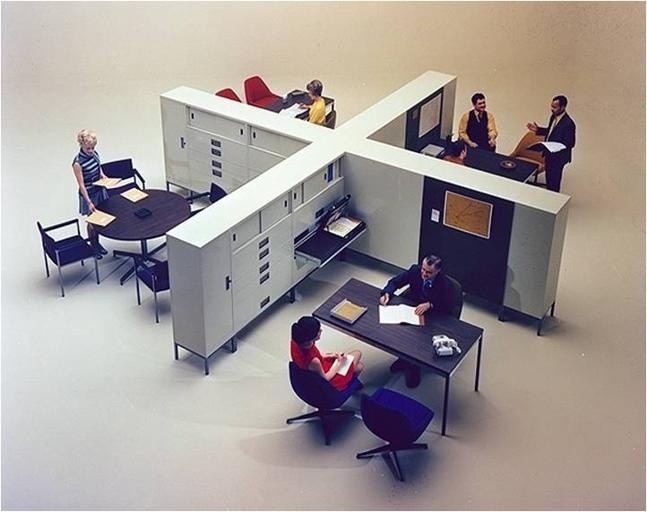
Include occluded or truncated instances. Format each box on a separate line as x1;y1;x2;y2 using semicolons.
478;111;482;121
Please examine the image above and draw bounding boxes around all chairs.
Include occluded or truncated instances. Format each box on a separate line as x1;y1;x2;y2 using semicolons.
98;158;145;198
134;255;170;323
286;362;360;445
37;219;100;297
356;389;434;481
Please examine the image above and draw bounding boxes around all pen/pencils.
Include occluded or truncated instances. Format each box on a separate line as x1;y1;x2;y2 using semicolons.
384;293;387;302
341;353;344;356
297;102;305;108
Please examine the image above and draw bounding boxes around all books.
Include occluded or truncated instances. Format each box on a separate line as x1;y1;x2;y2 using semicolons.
85;208;117;228
378;304;425;326
120;187;149;203
279;102;309;118
526;141;567;153
92;178;122;187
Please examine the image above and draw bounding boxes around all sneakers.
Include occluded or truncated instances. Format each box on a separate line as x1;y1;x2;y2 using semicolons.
89;243;108;259
391;358;422;389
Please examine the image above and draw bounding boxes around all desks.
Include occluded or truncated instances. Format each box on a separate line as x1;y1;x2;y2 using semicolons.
89;189;191;285
313;278;484;436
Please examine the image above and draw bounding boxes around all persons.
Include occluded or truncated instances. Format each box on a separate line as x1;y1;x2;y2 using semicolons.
71;129;108;259
443;141;468;165
298;79;327;126
458;93;498;152
527;95;576;192
378;254;456;388
290;316;365;394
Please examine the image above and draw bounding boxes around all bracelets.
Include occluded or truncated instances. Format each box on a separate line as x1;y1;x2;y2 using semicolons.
87;201;92;205
